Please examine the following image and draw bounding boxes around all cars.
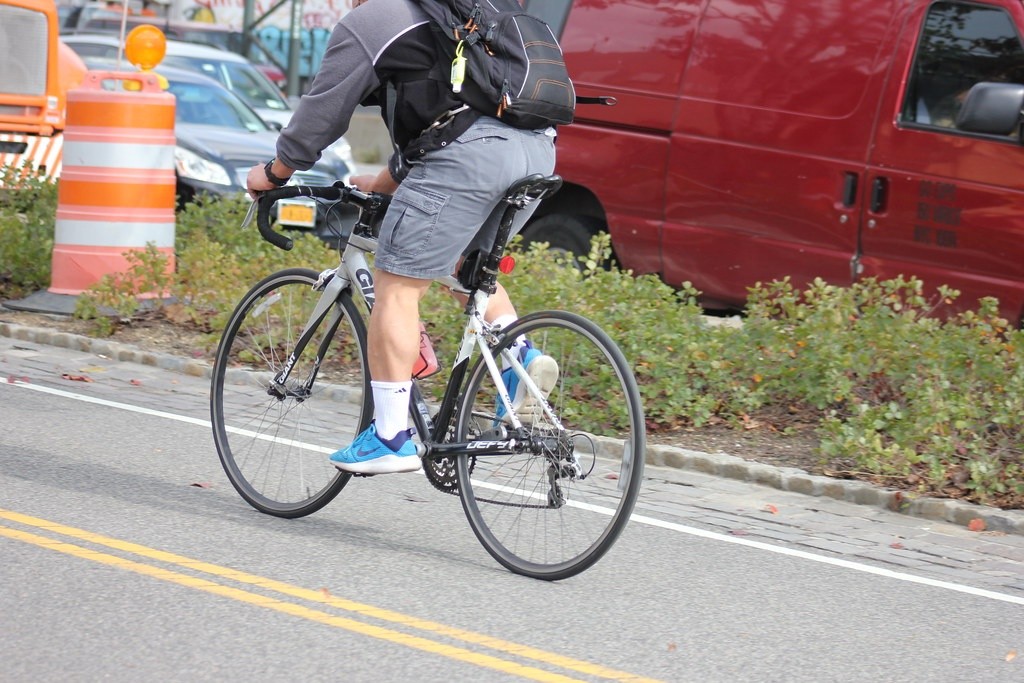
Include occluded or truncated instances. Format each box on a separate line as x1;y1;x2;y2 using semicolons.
56;17;353;213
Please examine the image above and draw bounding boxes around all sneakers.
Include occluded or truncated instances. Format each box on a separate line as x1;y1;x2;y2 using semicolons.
330;419;423;474
493;340;560;428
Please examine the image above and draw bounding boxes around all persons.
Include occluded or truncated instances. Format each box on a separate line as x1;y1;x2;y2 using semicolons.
247;1;559;474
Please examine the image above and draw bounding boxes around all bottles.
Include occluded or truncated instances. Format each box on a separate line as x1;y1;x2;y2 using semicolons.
412;314;437;379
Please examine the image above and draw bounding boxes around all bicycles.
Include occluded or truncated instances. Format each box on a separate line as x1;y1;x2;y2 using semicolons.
207;177;648;581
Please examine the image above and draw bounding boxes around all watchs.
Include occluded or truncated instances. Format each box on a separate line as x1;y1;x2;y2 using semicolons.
261;159;292;187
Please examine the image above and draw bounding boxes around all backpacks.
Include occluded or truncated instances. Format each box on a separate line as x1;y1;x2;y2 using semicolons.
417;0;577;129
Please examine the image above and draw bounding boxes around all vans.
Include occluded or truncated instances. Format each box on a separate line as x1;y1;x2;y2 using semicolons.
508;0;1024;336
